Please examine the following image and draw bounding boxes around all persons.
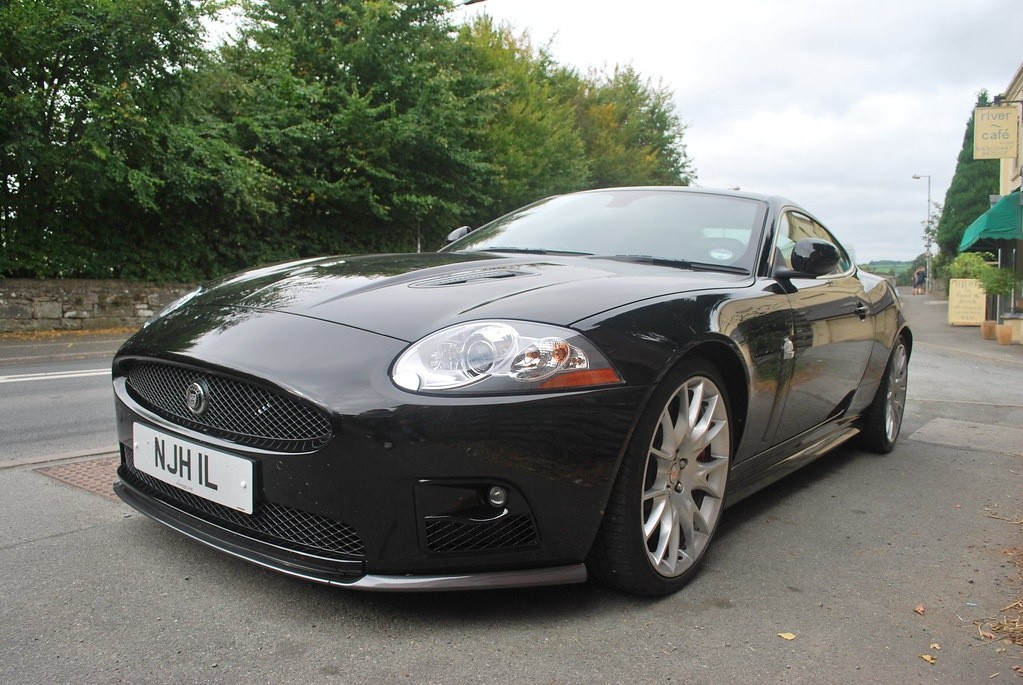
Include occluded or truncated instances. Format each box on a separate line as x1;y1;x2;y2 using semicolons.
912;266;926;295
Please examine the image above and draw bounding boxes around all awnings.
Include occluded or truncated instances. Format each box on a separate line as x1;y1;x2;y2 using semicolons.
959;191;1023;253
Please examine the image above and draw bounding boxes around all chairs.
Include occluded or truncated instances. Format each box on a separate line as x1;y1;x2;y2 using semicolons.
686;237;745;266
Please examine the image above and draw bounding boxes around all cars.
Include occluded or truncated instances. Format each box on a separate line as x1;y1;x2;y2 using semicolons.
108;184;914;602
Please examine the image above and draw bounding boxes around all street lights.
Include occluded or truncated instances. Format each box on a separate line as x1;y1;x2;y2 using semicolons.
409;0;485;254
911;174;933;295
